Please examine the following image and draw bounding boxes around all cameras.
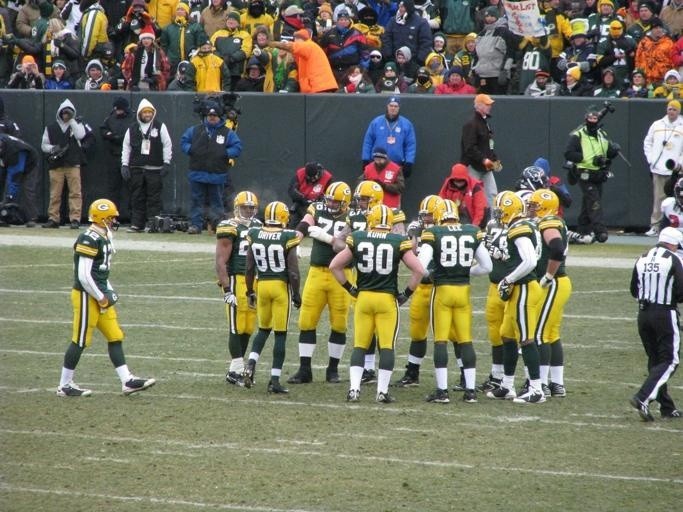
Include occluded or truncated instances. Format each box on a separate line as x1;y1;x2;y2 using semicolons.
666;159;683;177
132;9;144;17
288;199;302;215
592;154;612;167
47;148;65;164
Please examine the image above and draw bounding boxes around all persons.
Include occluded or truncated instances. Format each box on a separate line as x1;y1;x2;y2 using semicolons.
397;164;493;404
98;98;139;226
565;105;620;242
38;98;90;231
361;97;417;177
58;199;154;399
120;98;176;235
630;227;683;420
0;112;42;227
459;95;503;208
658;177;683;265
641;99;683;236
485;158;572;403
181;103;238;235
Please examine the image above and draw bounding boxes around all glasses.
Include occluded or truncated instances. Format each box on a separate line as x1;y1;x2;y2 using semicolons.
370;54;380;59
54;64;64;70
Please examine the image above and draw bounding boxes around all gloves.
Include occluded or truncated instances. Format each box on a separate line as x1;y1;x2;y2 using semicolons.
483;158;494;171
64;108;75;120
120;166;131;181
125;7;133;22
292;294;301;309
308;224;332;244
470;74;480;88
296;246;302;258
403;162;411;177
498;69;508;86
348;286;358;298
160;163;170;177
54;39;63;48
363;160;368;171
426;269;434;282
499;278;511;301
607;142;621;160
141;11;152;25
224;291;237;307
252;44;260;56
396;292;408;306
246;291;257;310
488;245;504;261
540;273;553;289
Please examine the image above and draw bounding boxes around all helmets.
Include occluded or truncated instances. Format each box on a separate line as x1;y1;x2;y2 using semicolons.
519;165;549;191
527;189;559;220
368;205;393;230
352;180;383;213
516;189;533;203
432;199;460;225
264;201;290;229
88;199;119;232
492;190;525;227
419;194;443;228
322;181;352;214
673;177;683;208
234;190;258;222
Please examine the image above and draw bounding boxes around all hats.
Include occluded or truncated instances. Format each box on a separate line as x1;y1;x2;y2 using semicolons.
39;1;52;17
474;94;495;105
253;24;270;41
337;9;348;17
598;0;615;7
638;0;656;13
603;67;614;74
52;59;66;71
668;99;681;110
179;63;186;72
569;20;593;39
372;147;388;159
200;35;210;46
435;36;444;43
384;95;400;105
303;162;319;177
228;8;240;20
448;64;463;76
650;18;662;29
294;28;309;39
630;69;643;79
383;62;396;73
112;96;130;113
584;105;599;119
416;65;429;78
284;5;304;16
485;5;499;18
140;108;152;114
176;0;190;14
139;23;155;40
567;65;581;80
132;0;145;7
207;108;219;116
535;65;549;76
659;226;683;249
22;54;34;64
318;2;333;13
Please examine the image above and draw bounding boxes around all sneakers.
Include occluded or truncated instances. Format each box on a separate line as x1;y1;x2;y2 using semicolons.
517;380;529;395
122;375;156;396
268;381;289;393
376;391;395;402
476;376;502;392
286;368;312;382
463;389;478;402
127;227;139;234
346;388;360;401
56;380;91;396
644;226;659;236
71;221;79;229
144;226;153;233
245;365;254;387
661;409;683;417
361;369;377;384
583;232;595;244
326;367;339;382
226;371;245;386
188;225;201;234
630;396;654;422
425;389;449;403
391;373;419;386
454;385;466;390
0;221;8;226
514;388;546;404
27;221;36;227
487;383;517;400
548;382;566;397
598;232;607;242
42;221;59;228
543;384;551;397
